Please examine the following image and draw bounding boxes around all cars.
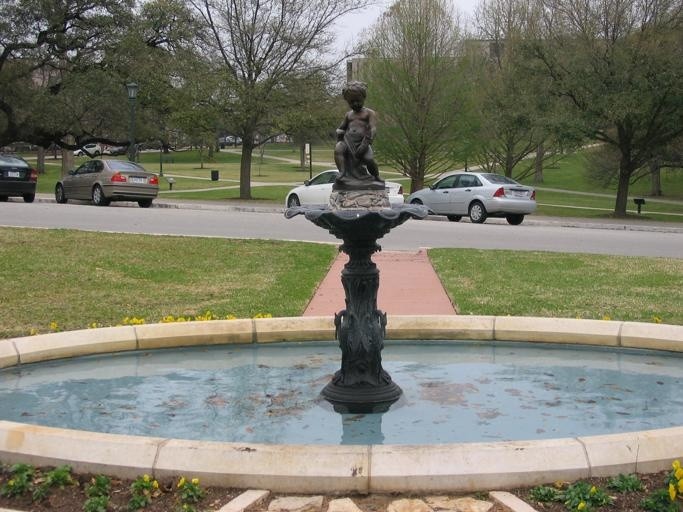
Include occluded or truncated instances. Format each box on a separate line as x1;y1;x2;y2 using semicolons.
284;170;406;215
216;135;242;146
406;172;536;224
71;139;171;158
0;154;39;201
54;159;160;209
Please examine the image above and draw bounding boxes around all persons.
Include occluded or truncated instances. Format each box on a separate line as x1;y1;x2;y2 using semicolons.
333;80;386;184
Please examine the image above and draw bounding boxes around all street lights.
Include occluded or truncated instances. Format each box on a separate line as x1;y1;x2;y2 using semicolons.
126;81;140;161
